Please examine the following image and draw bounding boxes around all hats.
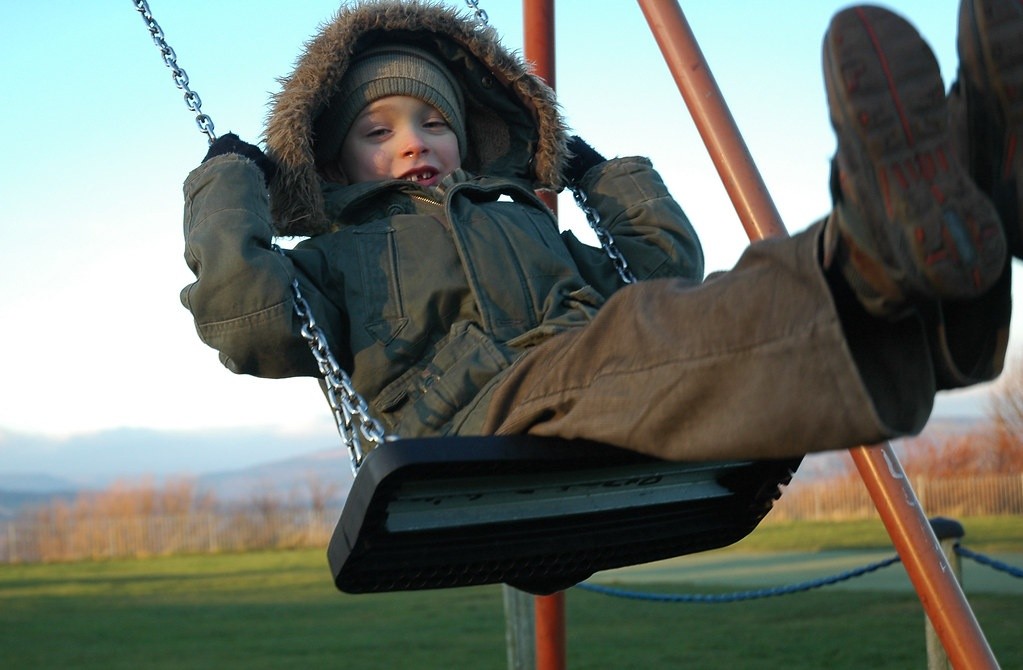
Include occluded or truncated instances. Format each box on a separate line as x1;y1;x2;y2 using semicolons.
313;44;468;170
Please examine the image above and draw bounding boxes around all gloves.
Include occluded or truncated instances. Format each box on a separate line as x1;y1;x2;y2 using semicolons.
200;133;278;190
555;136;607;193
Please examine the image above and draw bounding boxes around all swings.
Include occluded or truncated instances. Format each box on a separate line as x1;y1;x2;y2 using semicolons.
131;1;816;600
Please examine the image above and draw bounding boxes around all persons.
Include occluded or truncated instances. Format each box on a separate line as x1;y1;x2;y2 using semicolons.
179;1;1022;463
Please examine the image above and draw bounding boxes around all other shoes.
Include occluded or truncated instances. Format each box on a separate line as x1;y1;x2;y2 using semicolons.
957;0;1023;259
822;5;1006;306
